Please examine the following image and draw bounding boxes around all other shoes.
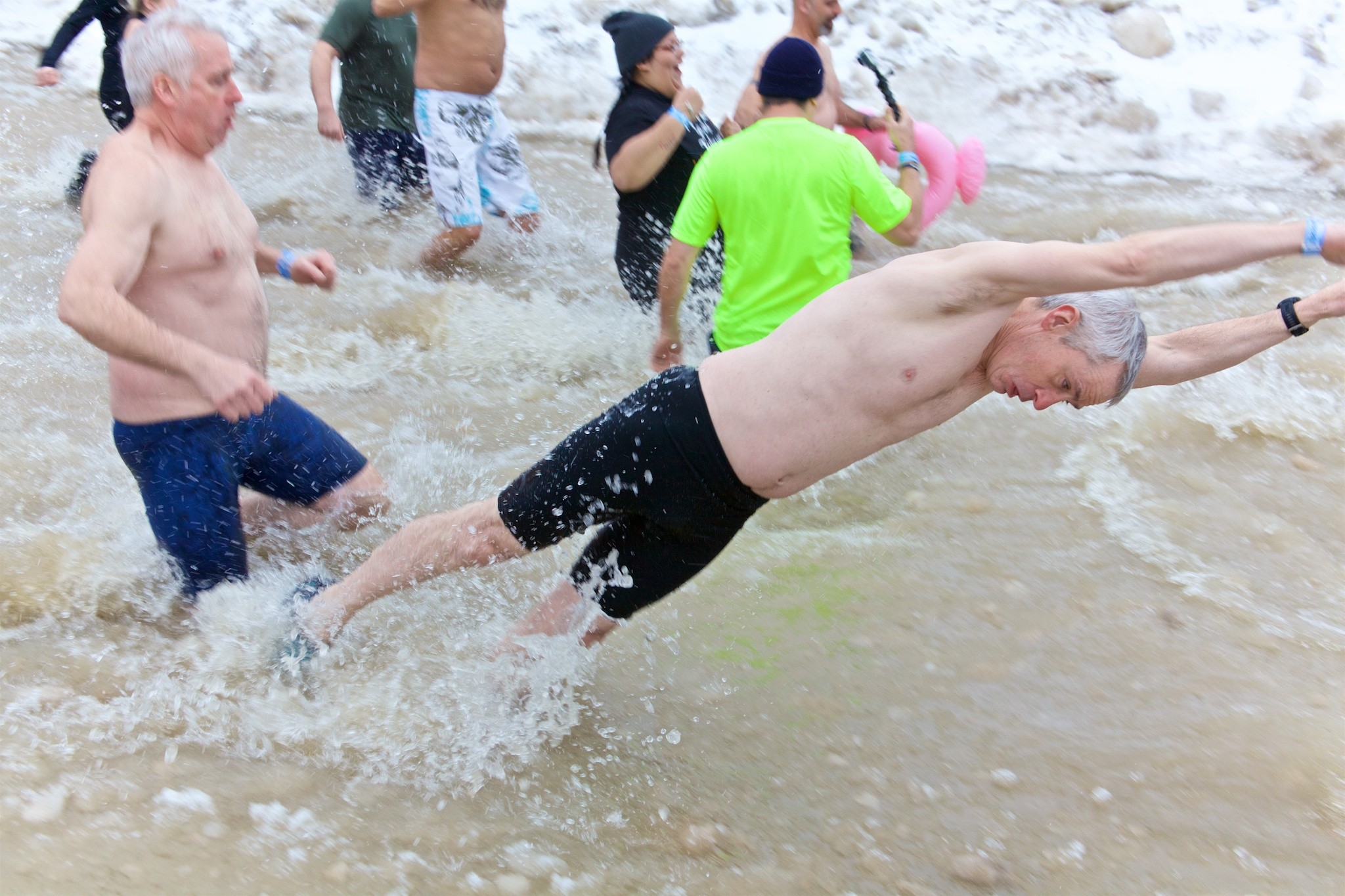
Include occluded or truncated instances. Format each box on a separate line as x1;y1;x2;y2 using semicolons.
281;579;331;671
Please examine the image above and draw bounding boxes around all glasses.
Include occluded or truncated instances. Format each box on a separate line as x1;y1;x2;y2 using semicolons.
652;38;682;55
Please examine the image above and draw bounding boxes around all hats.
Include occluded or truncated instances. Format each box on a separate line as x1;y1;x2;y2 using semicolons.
604;10;675;75
758;36;823;99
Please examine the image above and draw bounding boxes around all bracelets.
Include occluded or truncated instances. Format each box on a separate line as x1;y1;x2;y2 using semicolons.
277;249;299;280
898;151;920;165
1302;219;1328;258
666;106;690;130
899;161;920;171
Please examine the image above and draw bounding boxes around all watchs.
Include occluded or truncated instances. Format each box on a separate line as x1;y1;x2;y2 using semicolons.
1276;297;1309;337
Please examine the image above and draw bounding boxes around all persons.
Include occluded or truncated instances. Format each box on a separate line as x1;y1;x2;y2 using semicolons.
732;1;888;263
600;10;741;316
34;1;140;207
124;0;177;40
372;0;542;265
310;0;435;210
305;218;1345;691
651;34;924;375
59;2;390;596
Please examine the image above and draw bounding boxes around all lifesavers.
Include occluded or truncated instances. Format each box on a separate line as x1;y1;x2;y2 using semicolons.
843;105;988;229
